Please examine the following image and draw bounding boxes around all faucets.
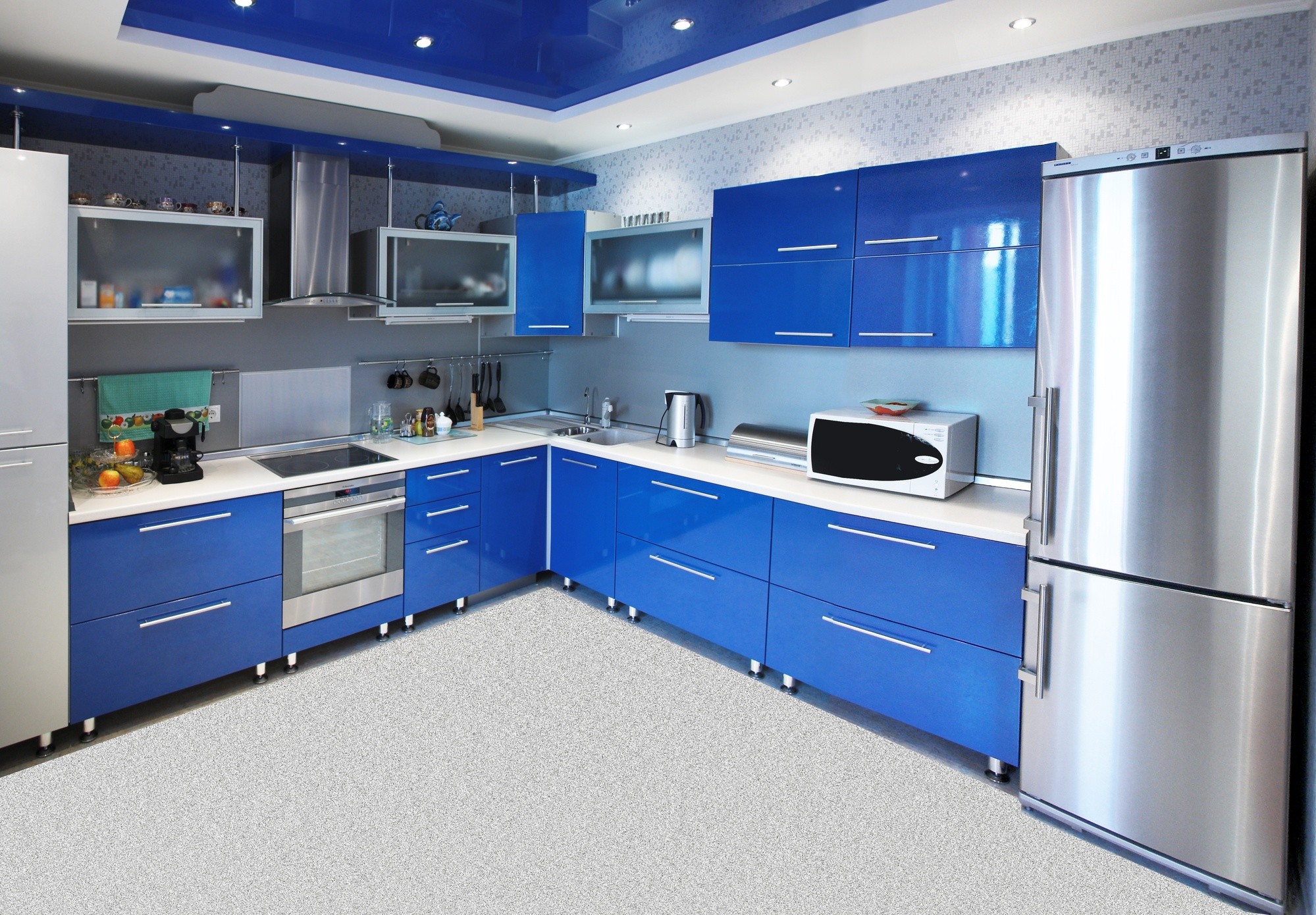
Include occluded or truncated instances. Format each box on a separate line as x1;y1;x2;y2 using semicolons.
583;387;593;425
600;396;614;430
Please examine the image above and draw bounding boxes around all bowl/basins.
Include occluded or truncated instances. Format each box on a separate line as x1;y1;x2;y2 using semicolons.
434;411;452;435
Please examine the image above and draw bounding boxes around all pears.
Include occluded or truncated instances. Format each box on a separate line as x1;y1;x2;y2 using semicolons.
114;463;144;484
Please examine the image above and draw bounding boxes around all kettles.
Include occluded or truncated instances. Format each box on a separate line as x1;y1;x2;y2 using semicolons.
665;392;706;448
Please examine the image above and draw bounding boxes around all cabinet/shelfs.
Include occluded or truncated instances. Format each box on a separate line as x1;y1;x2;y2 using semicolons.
68;442;547;730
708;138;1059;348
544;446;1020;771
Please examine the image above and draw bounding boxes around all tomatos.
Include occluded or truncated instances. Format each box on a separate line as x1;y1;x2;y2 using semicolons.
112;416;124;426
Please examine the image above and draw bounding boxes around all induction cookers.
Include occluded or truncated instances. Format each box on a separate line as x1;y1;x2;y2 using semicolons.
247;439;405;519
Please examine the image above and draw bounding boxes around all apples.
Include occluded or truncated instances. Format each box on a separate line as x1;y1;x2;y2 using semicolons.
115;439;136;457
98;470;120;490
124;417;134;428
186;410;202;419
101;419;112;429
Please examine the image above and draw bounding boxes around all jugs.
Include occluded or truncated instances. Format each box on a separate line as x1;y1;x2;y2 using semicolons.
368;401;394;443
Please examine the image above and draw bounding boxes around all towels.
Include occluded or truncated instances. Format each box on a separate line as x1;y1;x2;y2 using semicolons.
95;369;212;443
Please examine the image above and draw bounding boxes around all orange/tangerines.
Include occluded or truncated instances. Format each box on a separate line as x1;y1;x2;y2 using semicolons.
134;416;143;426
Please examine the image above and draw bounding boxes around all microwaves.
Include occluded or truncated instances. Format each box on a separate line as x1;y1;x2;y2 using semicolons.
808;405;976;499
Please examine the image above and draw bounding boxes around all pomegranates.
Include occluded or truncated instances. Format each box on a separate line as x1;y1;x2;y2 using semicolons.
201;407;208;417
152;413;163;421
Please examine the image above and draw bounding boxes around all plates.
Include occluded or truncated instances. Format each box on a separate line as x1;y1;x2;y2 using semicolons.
90;447;139;463
72;468;157;494
860;399;920;415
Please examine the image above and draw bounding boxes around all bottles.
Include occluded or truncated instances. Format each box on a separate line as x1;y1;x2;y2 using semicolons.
415;410;423;435
400;412;416;437
420;407;435;437
601;397;611;428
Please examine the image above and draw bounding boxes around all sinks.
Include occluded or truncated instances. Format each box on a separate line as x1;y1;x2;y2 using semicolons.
567;426;666;450
551;423;600;438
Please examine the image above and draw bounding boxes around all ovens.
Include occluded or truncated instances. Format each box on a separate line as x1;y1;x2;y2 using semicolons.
282;497;404;631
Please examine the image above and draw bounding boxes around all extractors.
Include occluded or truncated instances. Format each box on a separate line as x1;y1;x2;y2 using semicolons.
262;150;398;308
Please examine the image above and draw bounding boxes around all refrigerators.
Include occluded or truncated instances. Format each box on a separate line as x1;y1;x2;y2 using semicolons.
1017;131;1310;914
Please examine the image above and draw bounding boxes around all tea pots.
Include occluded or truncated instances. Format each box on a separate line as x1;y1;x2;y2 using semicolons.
414;200;461;231
168;443;205;472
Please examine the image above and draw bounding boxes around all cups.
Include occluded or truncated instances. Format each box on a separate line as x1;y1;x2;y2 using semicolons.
127;199;152;210
155;197;181;211
652;212;661;223
177;203;202;214
629;215;637;226
387;371;403;389
646;213;653;224
141;452;153;466
206;201;232;215
401;370;413;388
623;216;630;227
227;206;250;216
660;211;669;222
70;193;98;206
638;214;646;225
418;367;440;389
103;192;123;207
120;195;132;208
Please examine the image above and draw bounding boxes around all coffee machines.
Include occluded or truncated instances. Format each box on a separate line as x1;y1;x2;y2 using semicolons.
151;408;206;485
